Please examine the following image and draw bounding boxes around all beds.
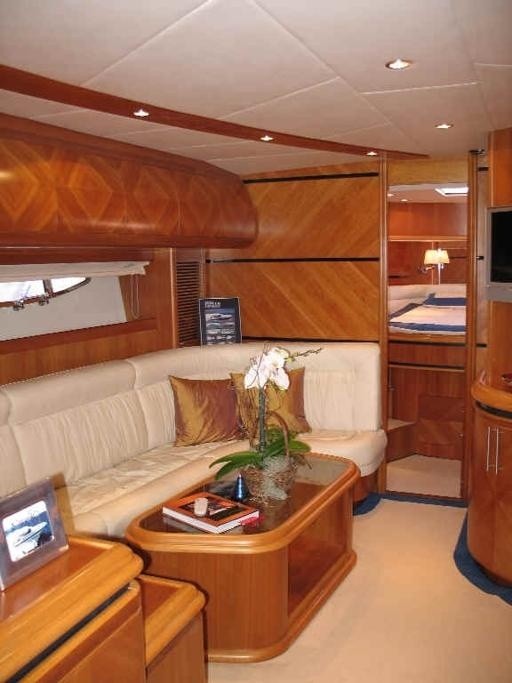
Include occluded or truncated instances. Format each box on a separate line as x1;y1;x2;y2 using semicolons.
388;282;467;345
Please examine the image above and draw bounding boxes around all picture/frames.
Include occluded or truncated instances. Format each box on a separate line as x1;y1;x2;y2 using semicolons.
0;475;69;594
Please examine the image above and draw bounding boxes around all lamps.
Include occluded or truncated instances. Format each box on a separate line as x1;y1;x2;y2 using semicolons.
416;244;451;275
0;274;94;310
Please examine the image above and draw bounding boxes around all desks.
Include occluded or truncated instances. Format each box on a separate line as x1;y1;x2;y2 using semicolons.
122;443;362;665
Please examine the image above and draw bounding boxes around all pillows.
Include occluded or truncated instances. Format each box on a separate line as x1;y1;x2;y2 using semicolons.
166;373;243;448
228;365;310;439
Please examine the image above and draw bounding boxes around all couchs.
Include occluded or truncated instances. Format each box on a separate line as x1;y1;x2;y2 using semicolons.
1;338;391;548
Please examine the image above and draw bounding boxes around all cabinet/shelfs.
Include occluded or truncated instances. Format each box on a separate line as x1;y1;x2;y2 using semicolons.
0;529;209;682
466;399;511;586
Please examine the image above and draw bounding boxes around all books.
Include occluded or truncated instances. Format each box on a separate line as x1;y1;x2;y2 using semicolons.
160;489;263;537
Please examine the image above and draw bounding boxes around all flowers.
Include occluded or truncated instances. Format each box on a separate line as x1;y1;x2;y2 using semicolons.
206;339;327;482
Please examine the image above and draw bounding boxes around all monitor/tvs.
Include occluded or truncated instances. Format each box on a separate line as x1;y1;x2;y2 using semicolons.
486;207;512;303
